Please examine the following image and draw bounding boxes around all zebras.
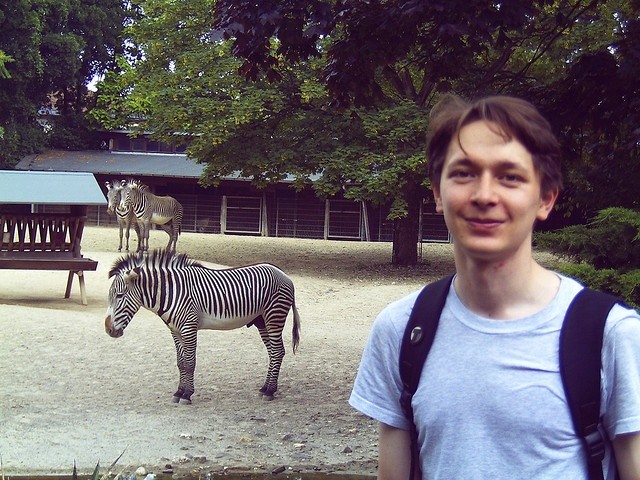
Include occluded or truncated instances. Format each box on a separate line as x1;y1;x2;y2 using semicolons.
106;180;156;252
105;247;301;406
120;178;184;253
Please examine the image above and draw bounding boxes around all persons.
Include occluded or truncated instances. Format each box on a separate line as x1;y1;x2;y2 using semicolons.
348;88;640;480
